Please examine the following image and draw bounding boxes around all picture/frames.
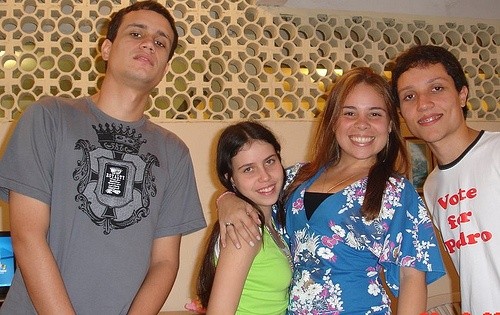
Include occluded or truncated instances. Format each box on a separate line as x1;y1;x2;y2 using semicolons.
405;136;434;196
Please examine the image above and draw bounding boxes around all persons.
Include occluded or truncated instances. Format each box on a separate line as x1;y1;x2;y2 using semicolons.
215;67;447;315
392;45;500;315
197;121;293;315
0;0;207;315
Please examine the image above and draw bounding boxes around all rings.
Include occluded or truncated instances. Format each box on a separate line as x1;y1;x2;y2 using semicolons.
226;223;234;226
246;209;256;216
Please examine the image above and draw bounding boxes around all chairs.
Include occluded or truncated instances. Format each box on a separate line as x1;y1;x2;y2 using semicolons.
380;226;462;315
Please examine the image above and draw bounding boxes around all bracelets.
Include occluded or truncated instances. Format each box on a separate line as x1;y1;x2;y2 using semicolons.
216;191;235;207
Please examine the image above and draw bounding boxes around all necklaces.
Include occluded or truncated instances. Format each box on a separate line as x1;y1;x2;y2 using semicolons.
265;225;294;273
322;167;360;193
